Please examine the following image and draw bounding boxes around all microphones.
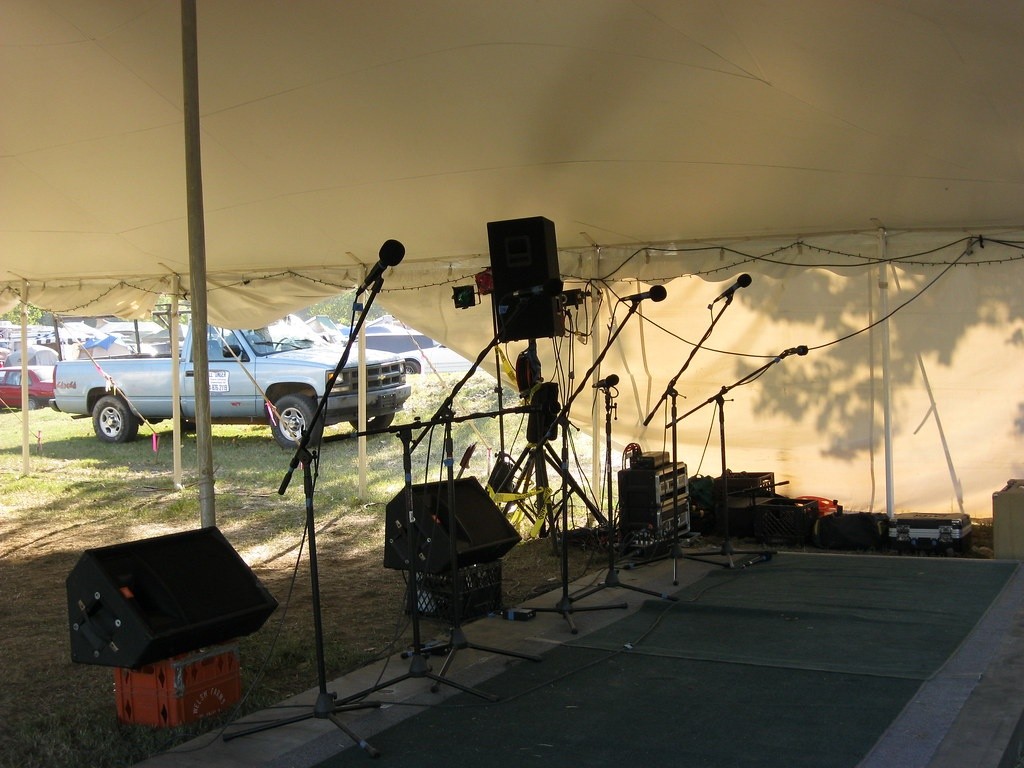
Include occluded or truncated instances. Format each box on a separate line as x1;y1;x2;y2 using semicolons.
620;285;666;302
593;374;619;388
356;239;405;296
508;279;564;297
784;346;808;355
712;274;752;301
519;401;560;415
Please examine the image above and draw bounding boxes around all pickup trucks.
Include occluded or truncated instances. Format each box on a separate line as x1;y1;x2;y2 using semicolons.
50;309;411;447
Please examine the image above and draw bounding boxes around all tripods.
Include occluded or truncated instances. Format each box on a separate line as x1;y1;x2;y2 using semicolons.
216;279;787;759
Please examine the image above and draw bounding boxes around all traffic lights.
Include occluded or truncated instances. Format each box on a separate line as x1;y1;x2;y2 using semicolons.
476;271;494;295
453;285;476;308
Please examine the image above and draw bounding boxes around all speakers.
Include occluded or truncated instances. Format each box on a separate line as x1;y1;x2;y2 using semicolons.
382;476;523;573
67;526;280;670
486;216;565;342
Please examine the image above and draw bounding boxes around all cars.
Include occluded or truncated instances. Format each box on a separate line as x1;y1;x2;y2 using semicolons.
0;366;57;411
399;335;483;372
